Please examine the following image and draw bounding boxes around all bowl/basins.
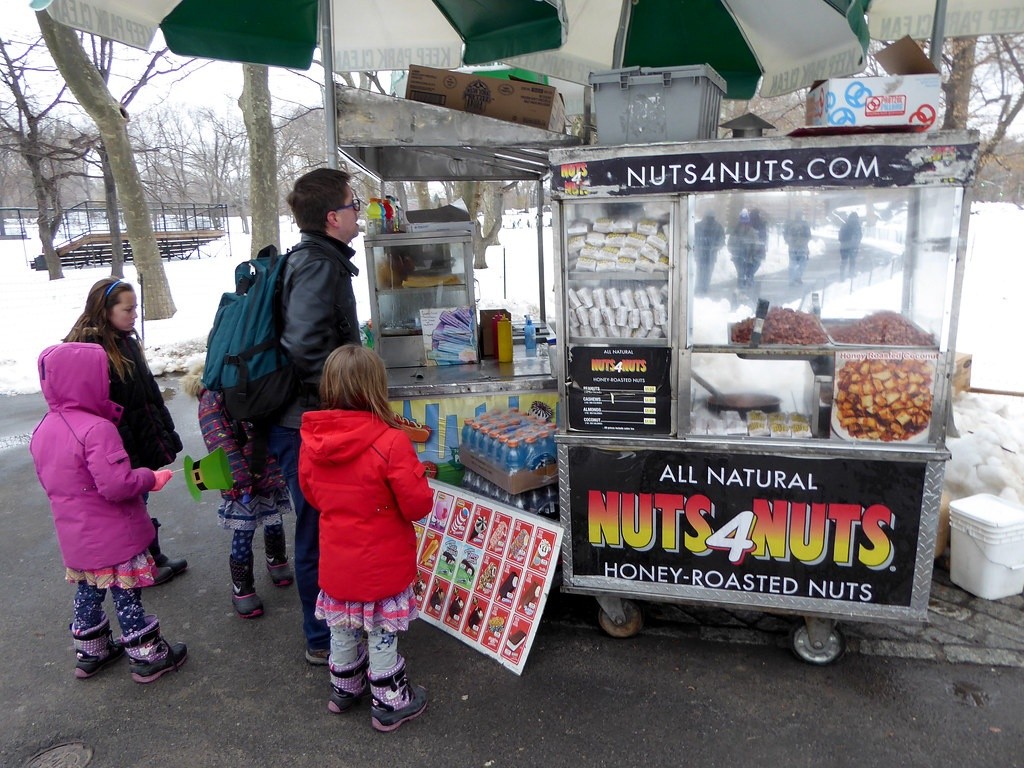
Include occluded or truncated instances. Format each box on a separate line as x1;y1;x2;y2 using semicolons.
707;393;781;418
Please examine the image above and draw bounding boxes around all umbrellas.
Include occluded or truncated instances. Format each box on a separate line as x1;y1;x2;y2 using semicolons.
861;0;1024;318
28;0;569;169
493;0;870;101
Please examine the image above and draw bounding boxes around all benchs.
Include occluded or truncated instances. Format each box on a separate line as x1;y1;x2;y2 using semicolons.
30;229;225;269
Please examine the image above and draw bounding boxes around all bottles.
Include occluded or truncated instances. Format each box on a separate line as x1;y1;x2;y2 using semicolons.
463;467;560;521
359;320;374;350
366;196;404;234
546;338;559;377
492;312;503;357
498;315;513;363
462;407;558;471
525;320;536;349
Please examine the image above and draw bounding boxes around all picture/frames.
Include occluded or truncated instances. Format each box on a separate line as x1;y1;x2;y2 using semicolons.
414;479;564;677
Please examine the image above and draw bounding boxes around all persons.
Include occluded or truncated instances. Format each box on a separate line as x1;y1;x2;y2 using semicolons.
29;342;189;683
727;207;766;290
783;209;812;287
62;278;188;587
838;212;863;283
263;169;369;676
297;344;434;732
695;211;725;295
183;359;294;619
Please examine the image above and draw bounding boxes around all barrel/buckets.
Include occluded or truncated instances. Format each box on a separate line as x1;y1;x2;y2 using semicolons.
949;493;1024;600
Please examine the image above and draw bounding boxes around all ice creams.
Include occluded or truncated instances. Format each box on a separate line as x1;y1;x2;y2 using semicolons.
469;515;487;541
531;539;552;565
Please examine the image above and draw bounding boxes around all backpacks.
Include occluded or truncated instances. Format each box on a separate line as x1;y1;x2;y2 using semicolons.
201;243;343;424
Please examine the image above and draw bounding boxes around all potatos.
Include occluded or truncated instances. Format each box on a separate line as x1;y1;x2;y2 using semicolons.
836;358;932;439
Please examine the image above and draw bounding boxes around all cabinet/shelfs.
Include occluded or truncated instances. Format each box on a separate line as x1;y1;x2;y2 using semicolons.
363;229;480;367
547;128;982;446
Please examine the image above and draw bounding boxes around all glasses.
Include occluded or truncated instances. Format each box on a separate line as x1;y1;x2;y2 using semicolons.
334;198;360;211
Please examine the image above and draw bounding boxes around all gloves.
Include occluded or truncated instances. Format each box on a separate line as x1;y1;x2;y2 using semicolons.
153;469;172;490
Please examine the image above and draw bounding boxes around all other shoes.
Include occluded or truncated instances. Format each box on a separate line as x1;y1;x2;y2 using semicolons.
305;647;328;664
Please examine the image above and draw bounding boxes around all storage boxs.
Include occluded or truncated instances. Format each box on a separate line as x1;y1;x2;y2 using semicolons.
405;62;595;134
588;63;727;147
805;34;939;128
459;445;558;497
481;309;511;359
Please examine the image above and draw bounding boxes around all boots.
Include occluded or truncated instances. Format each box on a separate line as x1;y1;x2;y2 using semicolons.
229;553;264;617
69;610;125;679
264;531;293;587
120;614;188;682
149;517;187;585
328;642;370;714
367;654;428;731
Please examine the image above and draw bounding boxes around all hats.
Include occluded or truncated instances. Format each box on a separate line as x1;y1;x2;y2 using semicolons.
736;208;751;223
